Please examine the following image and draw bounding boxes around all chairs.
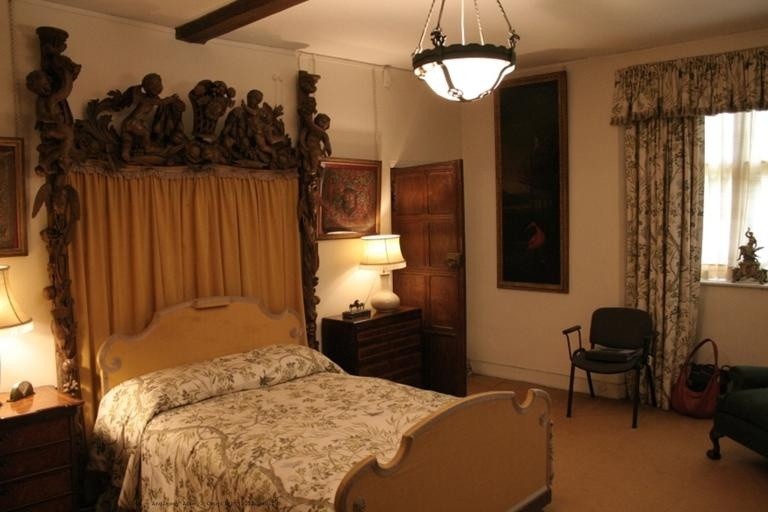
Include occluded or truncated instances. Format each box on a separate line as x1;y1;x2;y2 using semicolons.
562;307;656;428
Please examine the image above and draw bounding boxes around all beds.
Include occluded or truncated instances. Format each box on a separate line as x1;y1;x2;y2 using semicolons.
84;296;553;511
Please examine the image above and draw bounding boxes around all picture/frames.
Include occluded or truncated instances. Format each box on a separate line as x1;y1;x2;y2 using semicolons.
1;136;28;258
488;70;570;293
316;156;382;241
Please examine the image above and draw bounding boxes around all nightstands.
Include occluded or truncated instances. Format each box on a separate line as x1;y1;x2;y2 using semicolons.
322;306;423;390
0;384;85;510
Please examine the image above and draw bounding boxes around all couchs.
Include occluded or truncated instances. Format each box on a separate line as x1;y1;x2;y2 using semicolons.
707;365;768;461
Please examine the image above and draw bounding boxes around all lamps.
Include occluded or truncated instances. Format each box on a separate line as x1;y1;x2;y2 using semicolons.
359;235;406;311
0;265;33;330
412;0;520;103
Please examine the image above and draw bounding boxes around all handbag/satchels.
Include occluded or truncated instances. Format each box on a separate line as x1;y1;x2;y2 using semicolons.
670;337;722;421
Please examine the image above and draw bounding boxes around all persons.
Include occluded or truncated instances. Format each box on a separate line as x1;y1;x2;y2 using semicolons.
735;226;765;262
24;68;331;178
